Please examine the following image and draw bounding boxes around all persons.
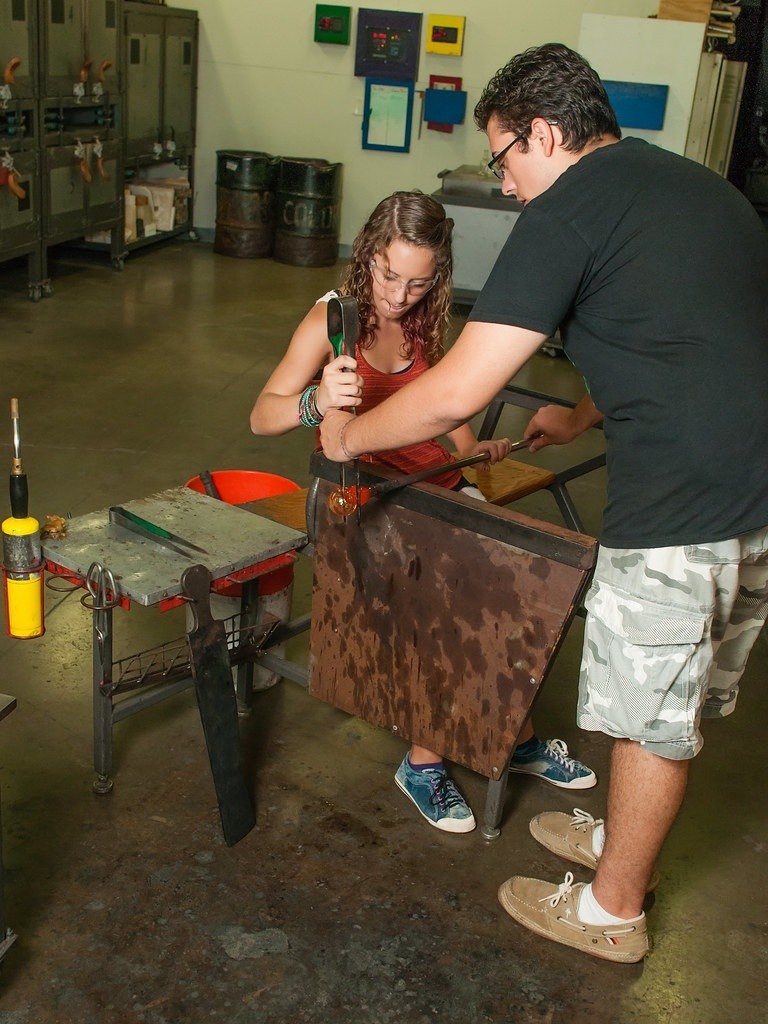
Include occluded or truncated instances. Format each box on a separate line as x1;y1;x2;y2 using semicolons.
250;190;597;832
319;44;768;963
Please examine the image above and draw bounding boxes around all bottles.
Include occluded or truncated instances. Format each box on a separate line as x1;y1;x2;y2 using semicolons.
480;149;490;176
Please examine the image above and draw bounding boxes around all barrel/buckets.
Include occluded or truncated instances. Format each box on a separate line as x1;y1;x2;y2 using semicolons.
213;149;276;259
273;155;343;268
185;471;304;693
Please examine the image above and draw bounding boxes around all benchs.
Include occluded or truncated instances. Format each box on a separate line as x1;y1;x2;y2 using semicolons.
229;449;551;718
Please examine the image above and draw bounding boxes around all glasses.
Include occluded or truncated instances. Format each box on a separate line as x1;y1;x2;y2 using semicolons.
369;258;442;296
487;120;558;179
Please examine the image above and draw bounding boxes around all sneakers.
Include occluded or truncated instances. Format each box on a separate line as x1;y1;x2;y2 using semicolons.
507;738;598;789
529;807;665;893
497;875;649;963
394;751;476;833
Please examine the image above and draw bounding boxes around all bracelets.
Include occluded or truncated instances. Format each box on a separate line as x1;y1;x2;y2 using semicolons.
297;386;324;428
341;418;362;459
